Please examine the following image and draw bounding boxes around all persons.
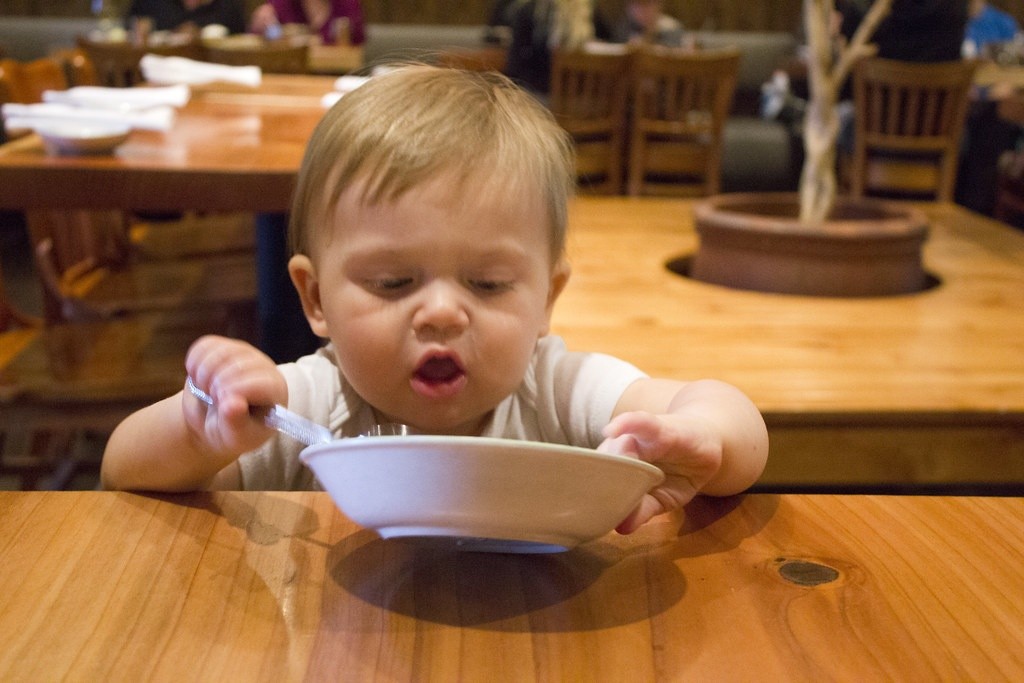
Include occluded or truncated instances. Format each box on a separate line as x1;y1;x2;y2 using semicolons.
952;0;1024;214
99;66;770;534
125;0;366;89
484;0;662;95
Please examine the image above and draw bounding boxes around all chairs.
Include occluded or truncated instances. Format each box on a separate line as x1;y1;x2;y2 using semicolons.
852;59;981;202
549;53;626;193
627;48;742;197
3;50;257;311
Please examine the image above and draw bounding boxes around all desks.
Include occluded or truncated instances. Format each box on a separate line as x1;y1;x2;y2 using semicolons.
0;73;369;365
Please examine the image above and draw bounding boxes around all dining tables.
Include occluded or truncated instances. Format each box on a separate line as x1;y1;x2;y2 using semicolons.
0;492;1024;683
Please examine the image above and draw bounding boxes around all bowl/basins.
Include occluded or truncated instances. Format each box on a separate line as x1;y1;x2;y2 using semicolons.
295;434;664;557
32;114;133;155
